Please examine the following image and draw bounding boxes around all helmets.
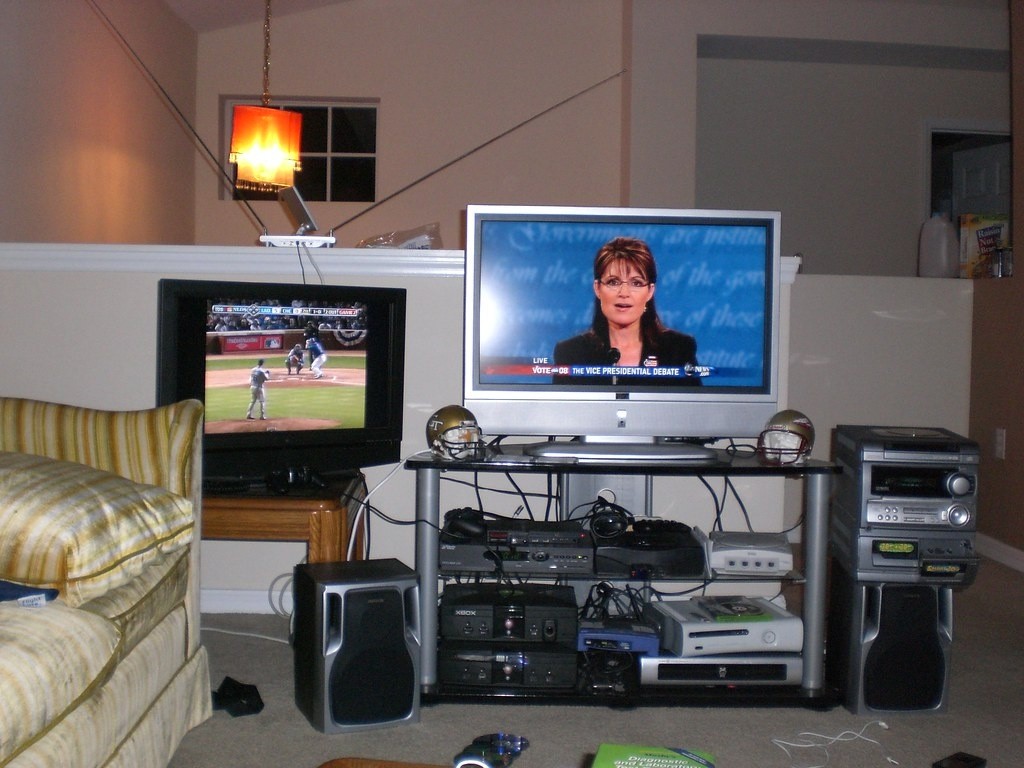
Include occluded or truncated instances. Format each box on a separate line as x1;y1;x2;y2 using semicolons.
426;405;487;462
757;410;815;466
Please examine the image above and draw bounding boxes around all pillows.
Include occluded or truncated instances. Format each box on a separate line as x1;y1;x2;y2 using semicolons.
0;452;194;608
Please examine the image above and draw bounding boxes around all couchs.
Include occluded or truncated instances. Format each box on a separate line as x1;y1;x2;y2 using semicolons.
0;395;214;768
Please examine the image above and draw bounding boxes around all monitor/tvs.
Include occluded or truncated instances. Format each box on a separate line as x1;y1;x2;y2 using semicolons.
465;204;790;460
155;278;407;496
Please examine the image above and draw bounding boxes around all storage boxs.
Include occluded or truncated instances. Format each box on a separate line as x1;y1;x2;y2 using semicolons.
592;744;717;768
959;213;1010;279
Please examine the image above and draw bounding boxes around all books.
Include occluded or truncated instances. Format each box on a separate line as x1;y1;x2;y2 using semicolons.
590;743;717;768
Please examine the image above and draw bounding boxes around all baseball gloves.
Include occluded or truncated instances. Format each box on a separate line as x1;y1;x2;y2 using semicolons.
291;355;298;366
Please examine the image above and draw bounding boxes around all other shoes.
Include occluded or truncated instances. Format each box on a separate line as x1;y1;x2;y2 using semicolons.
314;374;323;378
247;416;254;419
260;417;266;420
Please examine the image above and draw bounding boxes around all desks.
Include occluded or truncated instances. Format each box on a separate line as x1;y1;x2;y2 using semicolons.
200;472;366;564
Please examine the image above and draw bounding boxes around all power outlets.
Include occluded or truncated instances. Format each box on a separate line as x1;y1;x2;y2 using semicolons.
995;429;1005;458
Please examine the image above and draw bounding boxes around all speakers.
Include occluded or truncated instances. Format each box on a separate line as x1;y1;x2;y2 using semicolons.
292;557;421;734
825;560;954;714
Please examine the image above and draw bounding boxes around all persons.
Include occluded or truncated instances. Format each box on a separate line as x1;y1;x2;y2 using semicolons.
285;320;327;379
246;359;271;420
207;299;367;350
552;237;703;385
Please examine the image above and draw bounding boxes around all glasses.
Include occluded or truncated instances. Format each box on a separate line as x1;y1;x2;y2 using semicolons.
600;276;649;288
307;323;312;324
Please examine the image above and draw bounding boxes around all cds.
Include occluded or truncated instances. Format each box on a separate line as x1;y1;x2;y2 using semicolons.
453;734;529;768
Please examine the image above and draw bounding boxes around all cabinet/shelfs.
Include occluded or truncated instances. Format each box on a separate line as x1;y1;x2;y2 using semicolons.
408;442;833;711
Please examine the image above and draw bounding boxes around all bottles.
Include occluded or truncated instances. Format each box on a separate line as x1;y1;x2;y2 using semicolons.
1004;247;1012;278
919;211;960;277
993;247;1005;278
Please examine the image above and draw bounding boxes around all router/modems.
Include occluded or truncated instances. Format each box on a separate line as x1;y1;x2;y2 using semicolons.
90;0;628;248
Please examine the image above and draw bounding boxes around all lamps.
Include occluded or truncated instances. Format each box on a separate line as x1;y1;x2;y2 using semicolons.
229;0;302;193
